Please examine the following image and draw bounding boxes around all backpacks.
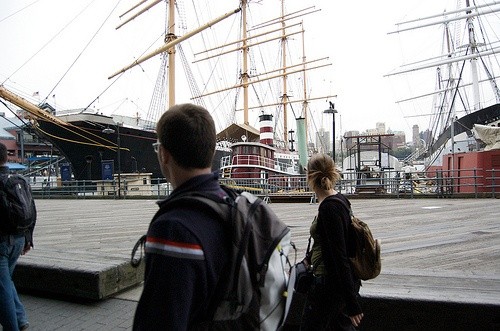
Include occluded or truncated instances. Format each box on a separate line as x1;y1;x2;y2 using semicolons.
326;195;381;281
130;185;297;331
0;170;37;237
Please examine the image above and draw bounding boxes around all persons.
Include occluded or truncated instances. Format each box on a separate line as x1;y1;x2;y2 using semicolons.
130;104;295;331
1;142;37;331
299;151;381;331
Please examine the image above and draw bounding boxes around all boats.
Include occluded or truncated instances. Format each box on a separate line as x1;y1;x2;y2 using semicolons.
0;0;500;193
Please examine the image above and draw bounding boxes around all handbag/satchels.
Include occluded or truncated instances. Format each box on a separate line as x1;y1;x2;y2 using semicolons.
284;257;316;331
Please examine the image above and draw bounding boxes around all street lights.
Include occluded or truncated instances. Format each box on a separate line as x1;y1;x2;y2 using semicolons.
102;121;121;196
322;101;338;163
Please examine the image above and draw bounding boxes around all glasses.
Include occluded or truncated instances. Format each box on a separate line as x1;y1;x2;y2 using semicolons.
152;142;161;152
